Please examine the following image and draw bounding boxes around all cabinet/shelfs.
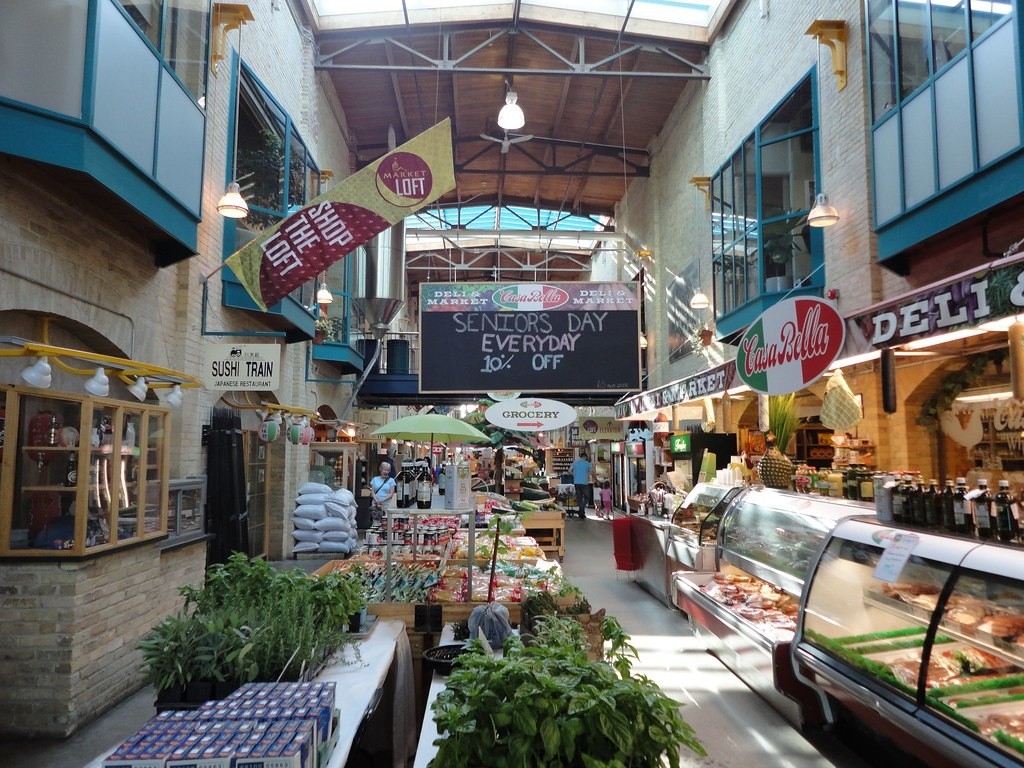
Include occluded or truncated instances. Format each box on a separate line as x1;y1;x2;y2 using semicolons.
22;447;161;492
552;452;573;471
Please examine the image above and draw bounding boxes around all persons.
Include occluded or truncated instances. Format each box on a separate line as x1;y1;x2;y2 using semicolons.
594;482;603;518
370;462;396;525
599;480;612;520
471;463;495;479
568;453;600;518
384;448;397;508
417;457;441;485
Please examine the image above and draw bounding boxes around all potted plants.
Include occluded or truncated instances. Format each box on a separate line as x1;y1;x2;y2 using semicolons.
424;498;708;768
758;391;808;489
311;317;343;344
135;550;361;713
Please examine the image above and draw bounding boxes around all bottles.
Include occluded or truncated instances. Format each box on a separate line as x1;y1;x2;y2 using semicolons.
995;479;1024;543
92;428;99;446
65;451;76;487
127;455;134;482
95;415;103;445
47;413;58;446
925;479;941;528
397;461;433;509
971;407;1024;461
912;479;926;526
940;479;956;531
891;479;905;523
553;449;573;470
104;415;112;445
972;479;997;540
795;462;886;504
953;477;972;535
902;476;914;524
125;422;135;447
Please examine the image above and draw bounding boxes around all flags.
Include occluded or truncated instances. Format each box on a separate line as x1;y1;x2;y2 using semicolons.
225;116;456;313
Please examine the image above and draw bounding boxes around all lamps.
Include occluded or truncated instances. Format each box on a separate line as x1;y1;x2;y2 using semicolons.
807;192;840;227
255;405;308;428
82;368;109;396
21;355;51;388
641;332;648;349
316;179;335;303
690;287;709;309
497;91;525;129
165;384;184;409
216;20;255;218
126;376;148;402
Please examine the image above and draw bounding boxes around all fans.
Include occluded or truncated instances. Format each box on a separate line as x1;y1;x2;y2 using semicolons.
480;129;533;153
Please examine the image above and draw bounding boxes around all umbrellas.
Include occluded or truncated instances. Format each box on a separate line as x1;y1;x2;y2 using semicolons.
371;414;492;494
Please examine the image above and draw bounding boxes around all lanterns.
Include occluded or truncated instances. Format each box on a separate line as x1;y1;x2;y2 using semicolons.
301;425;315;445
28;410;60;463
286;423;306;445
258;419;280;443
29;490;62;538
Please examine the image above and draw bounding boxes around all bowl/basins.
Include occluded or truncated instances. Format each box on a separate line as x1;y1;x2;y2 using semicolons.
421;644;483;675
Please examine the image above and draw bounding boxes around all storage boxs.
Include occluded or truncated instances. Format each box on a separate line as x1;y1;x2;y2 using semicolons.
101;681;343;768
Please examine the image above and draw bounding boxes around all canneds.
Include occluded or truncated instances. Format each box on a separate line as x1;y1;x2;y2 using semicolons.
789;464;921;502
365;516;451;557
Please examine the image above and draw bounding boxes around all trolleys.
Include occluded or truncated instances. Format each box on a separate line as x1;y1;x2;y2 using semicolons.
565;493;580;518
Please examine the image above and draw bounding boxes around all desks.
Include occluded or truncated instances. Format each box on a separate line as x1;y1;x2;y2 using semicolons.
83;617;407;768
412;621;520;768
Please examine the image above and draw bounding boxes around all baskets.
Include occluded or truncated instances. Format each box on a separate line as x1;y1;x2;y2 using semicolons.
422;644;469;675
471;477;488;504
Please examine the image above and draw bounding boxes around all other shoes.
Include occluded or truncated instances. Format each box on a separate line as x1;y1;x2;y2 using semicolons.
577;511;586;519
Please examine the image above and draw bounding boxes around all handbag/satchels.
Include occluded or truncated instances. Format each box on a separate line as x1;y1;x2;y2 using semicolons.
369;505;383;520
469;602;517;649
